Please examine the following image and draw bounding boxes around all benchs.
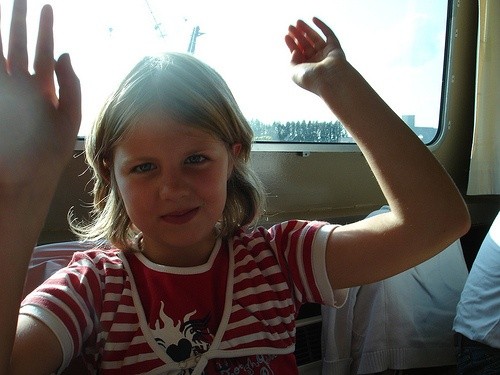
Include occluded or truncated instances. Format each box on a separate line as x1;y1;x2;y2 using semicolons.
244;194;500;375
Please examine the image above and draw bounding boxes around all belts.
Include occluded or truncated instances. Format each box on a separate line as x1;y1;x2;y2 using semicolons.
454;333;482;346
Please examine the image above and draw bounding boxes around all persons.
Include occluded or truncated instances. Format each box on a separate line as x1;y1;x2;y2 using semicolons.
451;209;500;375
0;0;470;375
